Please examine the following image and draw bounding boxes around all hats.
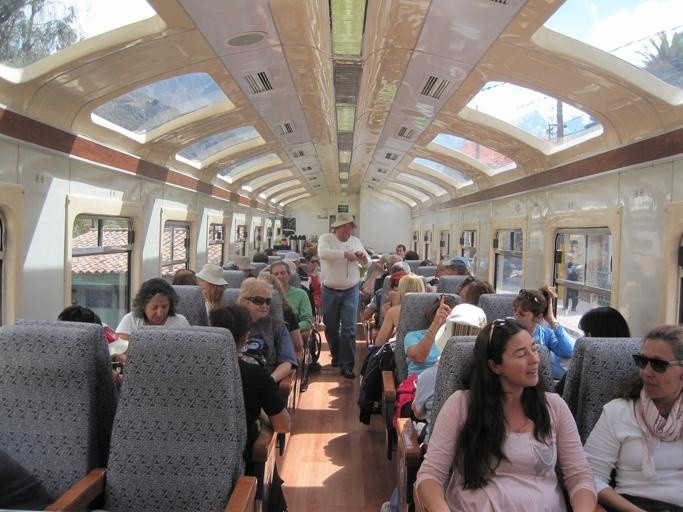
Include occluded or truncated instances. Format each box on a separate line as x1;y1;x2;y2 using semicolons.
233;255;257;270
390;271;408;282
194;263;229;286
442;256;470;270
281;251;299;262
102;322;129;356
434;303;488;354
330;212;358;229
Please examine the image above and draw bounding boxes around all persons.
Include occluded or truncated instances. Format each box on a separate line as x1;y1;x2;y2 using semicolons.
57;236;321;511
359;243;632;511
316;212;373;376
583;324;681;512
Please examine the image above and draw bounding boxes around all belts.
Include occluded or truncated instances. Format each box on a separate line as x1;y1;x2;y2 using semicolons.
321;284;358;293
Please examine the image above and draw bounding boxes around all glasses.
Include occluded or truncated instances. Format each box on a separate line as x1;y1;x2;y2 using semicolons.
519;289;542;306
631;352;682;373
488;316;517;352
391;279;399;289
243;296;272;306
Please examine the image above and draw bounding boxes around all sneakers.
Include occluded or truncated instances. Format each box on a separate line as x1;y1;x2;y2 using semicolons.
340;369;355;379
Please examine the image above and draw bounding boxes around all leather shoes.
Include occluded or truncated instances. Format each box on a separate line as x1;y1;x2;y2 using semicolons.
332;357;337;367
